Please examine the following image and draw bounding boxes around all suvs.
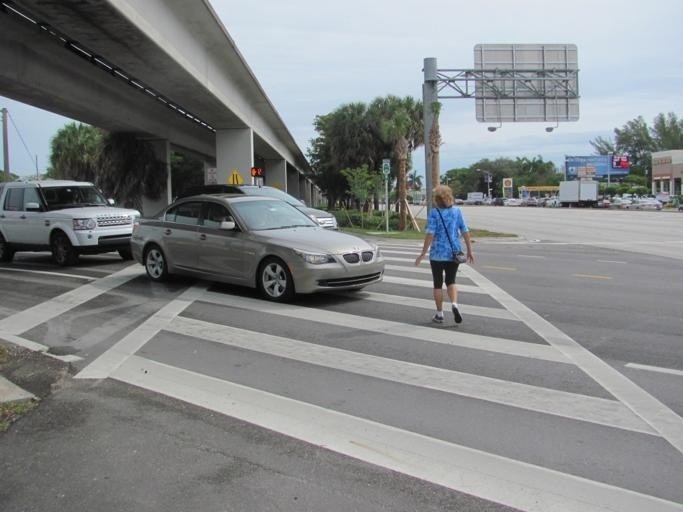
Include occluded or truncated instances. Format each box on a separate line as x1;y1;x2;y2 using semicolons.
173;184;338;229
0;179;142;268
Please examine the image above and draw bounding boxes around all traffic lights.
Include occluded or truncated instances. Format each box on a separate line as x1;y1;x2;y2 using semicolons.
250;167;264;177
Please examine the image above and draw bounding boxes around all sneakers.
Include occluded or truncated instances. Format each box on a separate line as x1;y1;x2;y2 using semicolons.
452;304;463;323
432;314;444;323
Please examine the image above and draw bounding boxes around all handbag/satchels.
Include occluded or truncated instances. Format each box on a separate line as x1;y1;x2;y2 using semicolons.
453;251;466;264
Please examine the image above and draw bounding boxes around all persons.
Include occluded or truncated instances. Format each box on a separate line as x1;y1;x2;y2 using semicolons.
415;184;475;324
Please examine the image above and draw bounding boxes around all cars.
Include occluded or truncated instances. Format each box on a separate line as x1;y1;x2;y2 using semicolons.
127;192;385;303
453;190;673;210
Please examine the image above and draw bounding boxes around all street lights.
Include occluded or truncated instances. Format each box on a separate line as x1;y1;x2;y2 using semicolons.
606;151;614;186
476;169;490;197
